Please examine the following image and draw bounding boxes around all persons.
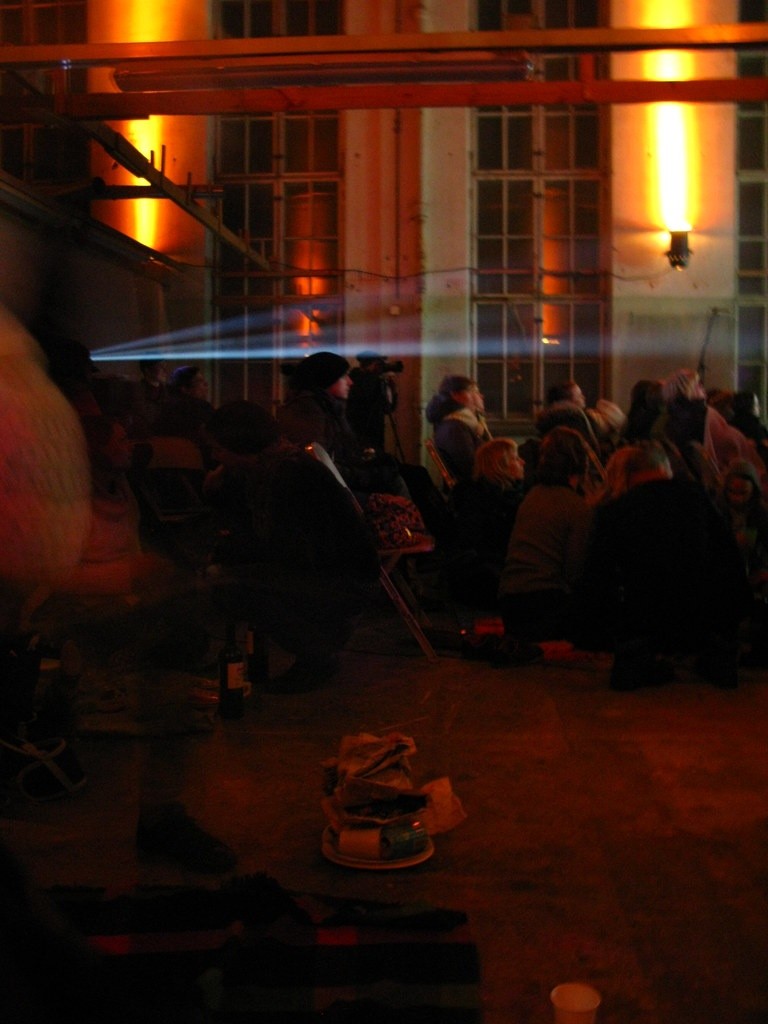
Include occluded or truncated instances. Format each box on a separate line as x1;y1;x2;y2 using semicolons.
72;342;768;876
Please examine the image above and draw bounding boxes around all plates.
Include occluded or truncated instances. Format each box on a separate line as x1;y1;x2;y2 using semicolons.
320;834;436;870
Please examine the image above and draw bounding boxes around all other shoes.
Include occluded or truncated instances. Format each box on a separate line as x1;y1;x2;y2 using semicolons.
302;617;353;657
266;651;340;695
496;636;543;666
462;633;501;662
698;636;738;689
607;657;676;690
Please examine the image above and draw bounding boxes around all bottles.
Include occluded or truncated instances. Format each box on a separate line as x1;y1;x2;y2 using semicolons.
219;619;247;719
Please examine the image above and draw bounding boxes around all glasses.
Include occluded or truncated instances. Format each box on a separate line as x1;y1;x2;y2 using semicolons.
190;376;206;384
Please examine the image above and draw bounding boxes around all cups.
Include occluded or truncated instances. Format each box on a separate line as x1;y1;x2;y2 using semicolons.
546;981;602;1024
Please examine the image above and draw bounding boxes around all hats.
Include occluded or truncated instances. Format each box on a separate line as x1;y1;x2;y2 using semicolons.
167;365;199;398
287;352;349;391
658;370;700;403
206;398;280;456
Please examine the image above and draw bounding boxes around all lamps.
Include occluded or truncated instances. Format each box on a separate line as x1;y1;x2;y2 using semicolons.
664;231;694;273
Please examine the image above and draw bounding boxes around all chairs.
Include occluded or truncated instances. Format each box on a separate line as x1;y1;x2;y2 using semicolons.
301;443;443;665
